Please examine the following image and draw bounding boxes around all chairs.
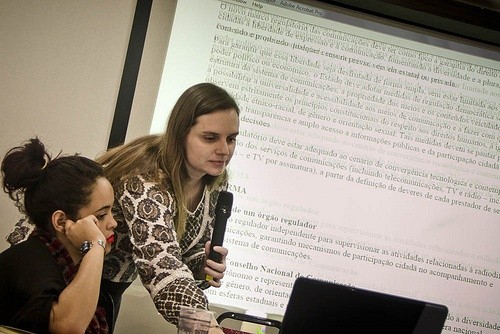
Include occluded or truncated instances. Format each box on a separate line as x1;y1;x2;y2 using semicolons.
218;277;449;334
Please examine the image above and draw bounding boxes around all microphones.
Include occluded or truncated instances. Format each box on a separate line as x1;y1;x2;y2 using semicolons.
205;191;233;282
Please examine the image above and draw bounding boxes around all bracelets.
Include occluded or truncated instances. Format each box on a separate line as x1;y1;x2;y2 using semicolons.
210;324;223;331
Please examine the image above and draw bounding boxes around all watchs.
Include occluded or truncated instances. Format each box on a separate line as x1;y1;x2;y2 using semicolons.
80;240;107;255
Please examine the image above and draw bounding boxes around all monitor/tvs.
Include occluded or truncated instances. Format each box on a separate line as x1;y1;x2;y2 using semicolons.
275;276;449;334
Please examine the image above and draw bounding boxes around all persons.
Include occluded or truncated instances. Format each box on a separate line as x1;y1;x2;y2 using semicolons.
94;83;240;334
0;137;118;334
